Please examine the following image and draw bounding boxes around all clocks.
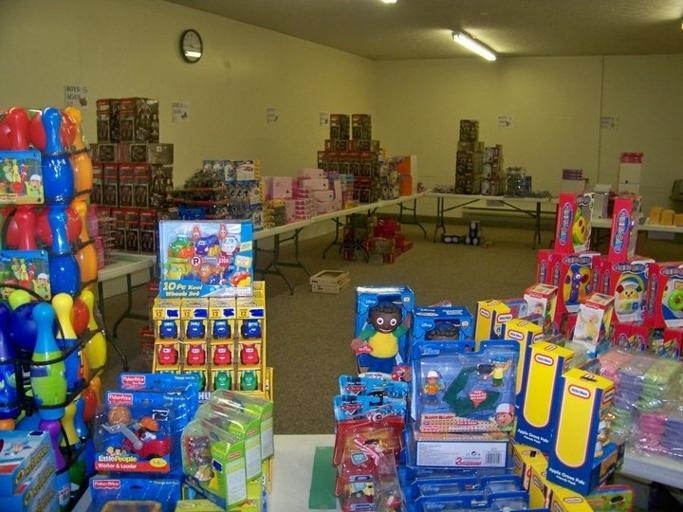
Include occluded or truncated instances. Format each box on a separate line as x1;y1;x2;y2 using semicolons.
179;30;204;64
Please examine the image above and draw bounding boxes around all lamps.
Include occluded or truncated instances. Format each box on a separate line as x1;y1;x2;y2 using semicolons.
451;31;497;64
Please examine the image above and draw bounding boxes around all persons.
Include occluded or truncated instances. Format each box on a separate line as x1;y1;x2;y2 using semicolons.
351;301;411;377
424;370;445;404
